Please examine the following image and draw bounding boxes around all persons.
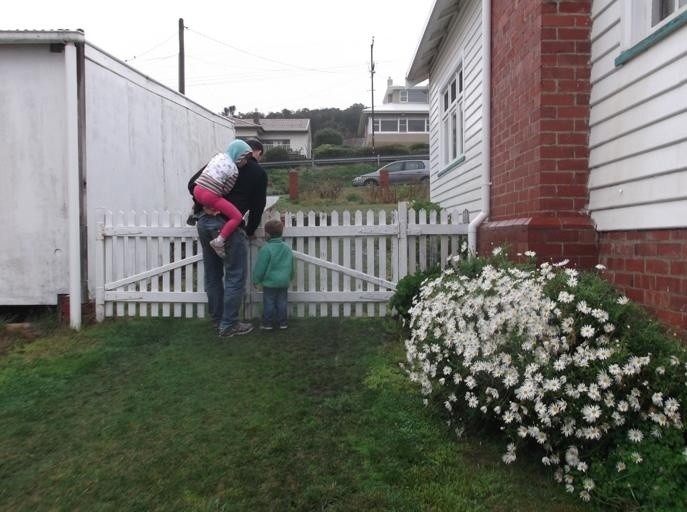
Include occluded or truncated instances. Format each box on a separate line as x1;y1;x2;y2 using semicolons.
251;221;295;331
189;140;268;337
187;139;253;259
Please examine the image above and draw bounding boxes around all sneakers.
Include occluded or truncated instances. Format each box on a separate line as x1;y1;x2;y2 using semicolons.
209;238;225;259
259;322;272;330
186;208;196;226
211;319;220;330
279;322;287;329
217;322;254;338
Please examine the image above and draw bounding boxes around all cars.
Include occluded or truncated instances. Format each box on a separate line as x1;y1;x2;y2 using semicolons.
352;160;430;187
